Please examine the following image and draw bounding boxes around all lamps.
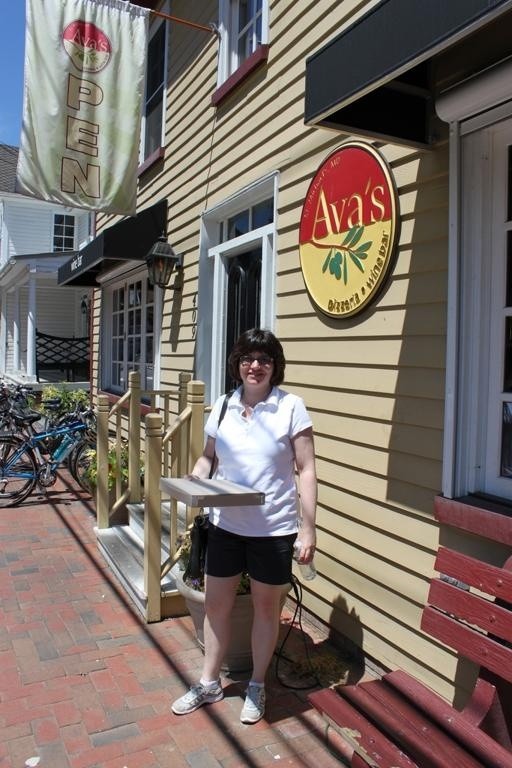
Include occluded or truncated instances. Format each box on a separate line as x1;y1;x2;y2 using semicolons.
145;228;184;291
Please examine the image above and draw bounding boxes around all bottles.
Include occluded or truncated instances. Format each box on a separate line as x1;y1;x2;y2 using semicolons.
293;540;317;582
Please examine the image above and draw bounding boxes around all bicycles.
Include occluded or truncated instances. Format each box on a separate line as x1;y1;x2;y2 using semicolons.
0;384;129;508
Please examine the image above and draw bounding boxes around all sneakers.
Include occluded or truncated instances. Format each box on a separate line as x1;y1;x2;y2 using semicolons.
172;678;224;715
240;685;266;724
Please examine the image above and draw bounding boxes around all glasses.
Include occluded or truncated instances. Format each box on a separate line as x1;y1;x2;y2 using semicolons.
241;355;274;366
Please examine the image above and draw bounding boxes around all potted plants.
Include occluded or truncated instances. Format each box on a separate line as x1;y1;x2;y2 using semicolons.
174;514;298;673
84;439;146;525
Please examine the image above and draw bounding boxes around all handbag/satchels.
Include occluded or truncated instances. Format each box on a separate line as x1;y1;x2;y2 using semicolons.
186;514;209;577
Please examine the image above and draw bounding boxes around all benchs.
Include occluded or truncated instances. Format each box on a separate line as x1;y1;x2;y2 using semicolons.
307;495;512;768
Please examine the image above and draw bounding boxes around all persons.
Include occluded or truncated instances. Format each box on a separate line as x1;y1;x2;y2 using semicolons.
170;327;319;724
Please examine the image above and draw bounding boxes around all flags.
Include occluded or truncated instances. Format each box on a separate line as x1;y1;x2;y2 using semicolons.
14;0;152;219
